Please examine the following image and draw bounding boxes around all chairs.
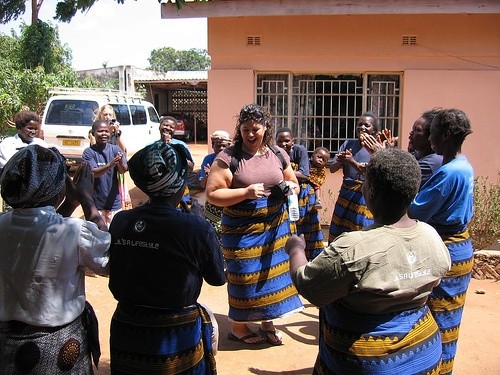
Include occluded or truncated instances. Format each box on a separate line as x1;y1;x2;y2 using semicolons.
59;110;83;124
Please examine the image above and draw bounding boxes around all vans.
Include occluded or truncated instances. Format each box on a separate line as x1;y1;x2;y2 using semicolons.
39;87;164;171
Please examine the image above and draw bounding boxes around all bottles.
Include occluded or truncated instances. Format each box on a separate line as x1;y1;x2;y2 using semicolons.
287;185;299;221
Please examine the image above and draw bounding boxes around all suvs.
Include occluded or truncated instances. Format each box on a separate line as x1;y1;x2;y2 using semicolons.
160;112;205;142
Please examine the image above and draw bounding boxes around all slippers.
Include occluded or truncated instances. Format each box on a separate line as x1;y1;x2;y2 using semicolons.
228;330;266;343
259;326;283;346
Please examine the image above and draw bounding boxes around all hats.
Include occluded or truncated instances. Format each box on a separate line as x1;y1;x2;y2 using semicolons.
0;145;67;209
127;140;192;200
210;131;232;141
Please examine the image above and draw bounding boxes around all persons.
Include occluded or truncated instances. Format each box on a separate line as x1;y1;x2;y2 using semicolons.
285;146;452;375
82;105;126;229
327;110;377;246
0;145;109;375
275;128;330;263
199;130;231;263
159;116;195;171
206;105;305;346
109;142;227;375
361;107;475;375
0;108;52;170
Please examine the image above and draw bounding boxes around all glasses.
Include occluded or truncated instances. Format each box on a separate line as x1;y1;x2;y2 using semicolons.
239;110;264;119
